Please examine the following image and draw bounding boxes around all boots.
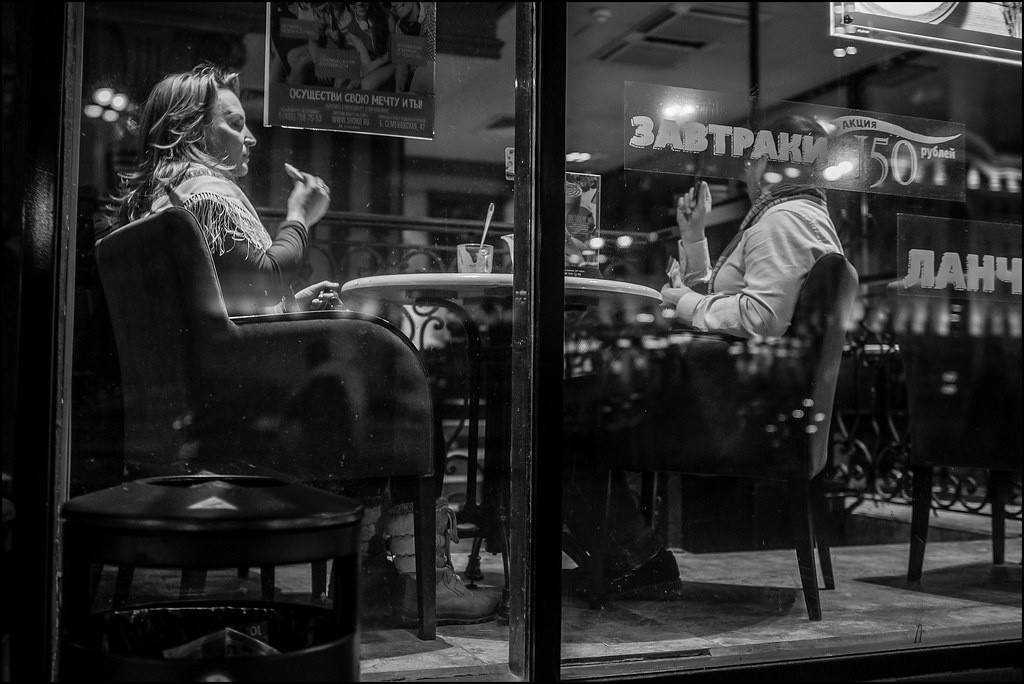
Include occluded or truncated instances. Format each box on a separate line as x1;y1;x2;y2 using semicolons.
385;492;506;628
346;485;412;629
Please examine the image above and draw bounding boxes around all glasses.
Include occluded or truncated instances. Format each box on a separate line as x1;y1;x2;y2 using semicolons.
740;145;767;171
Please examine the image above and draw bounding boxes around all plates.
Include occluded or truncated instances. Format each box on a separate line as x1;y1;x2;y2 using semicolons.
854;2;959;25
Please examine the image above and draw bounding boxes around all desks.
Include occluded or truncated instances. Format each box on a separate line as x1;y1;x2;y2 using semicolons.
342;274;663;625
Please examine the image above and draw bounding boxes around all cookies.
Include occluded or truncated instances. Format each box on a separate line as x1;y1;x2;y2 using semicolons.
284;163;305;182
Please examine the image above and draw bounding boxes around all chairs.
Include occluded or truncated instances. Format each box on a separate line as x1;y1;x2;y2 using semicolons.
581;252;860;621
889;280;1024;584
93;207;442;641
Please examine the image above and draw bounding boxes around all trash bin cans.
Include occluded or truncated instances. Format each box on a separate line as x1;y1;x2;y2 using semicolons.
60;466;369;684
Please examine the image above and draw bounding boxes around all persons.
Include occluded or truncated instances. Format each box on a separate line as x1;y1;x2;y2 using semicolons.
97;65;500;627
563;114;845;600
285;2;436;95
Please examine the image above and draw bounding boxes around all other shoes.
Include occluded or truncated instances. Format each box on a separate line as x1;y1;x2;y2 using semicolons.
582;543;684;607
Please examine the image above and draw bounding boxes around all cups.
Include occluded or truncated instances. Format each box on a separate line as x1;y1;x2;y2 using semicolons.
501;233;514;273
456;243;494;273
565;183;582;216
